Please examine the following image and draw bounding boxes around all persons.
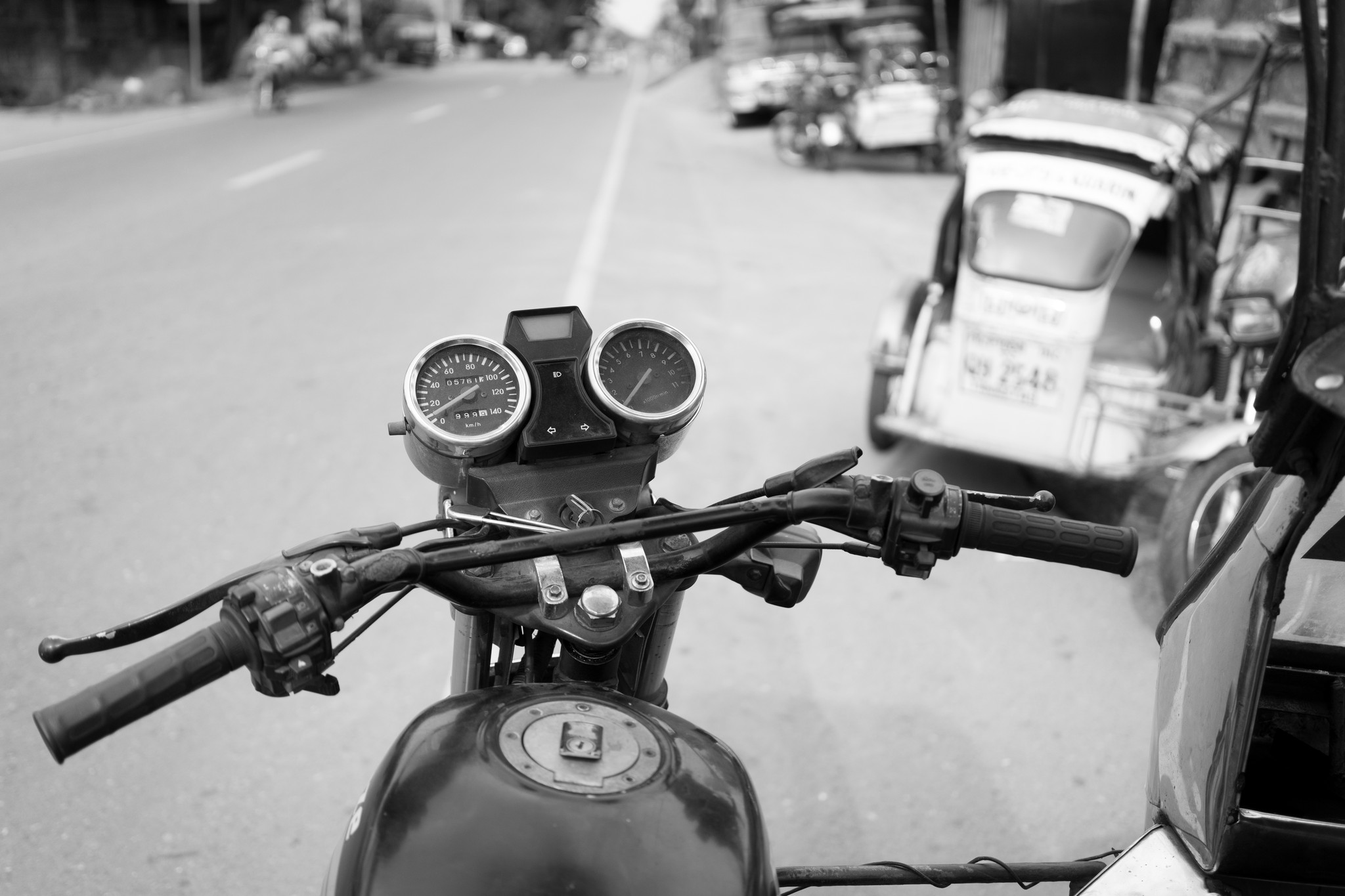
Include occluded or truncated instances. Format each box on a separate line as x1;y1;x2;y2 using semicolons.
243;6;313;109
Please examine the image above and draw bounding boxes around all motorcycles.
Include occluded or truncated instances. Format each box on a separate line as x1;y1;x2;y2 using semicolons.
248;40;293;109
24;291;1148;896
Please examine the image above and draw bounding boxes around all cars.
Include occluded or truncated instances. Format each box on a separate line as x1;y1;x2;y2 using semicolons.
860;86;1219;539
725;3;953;172
